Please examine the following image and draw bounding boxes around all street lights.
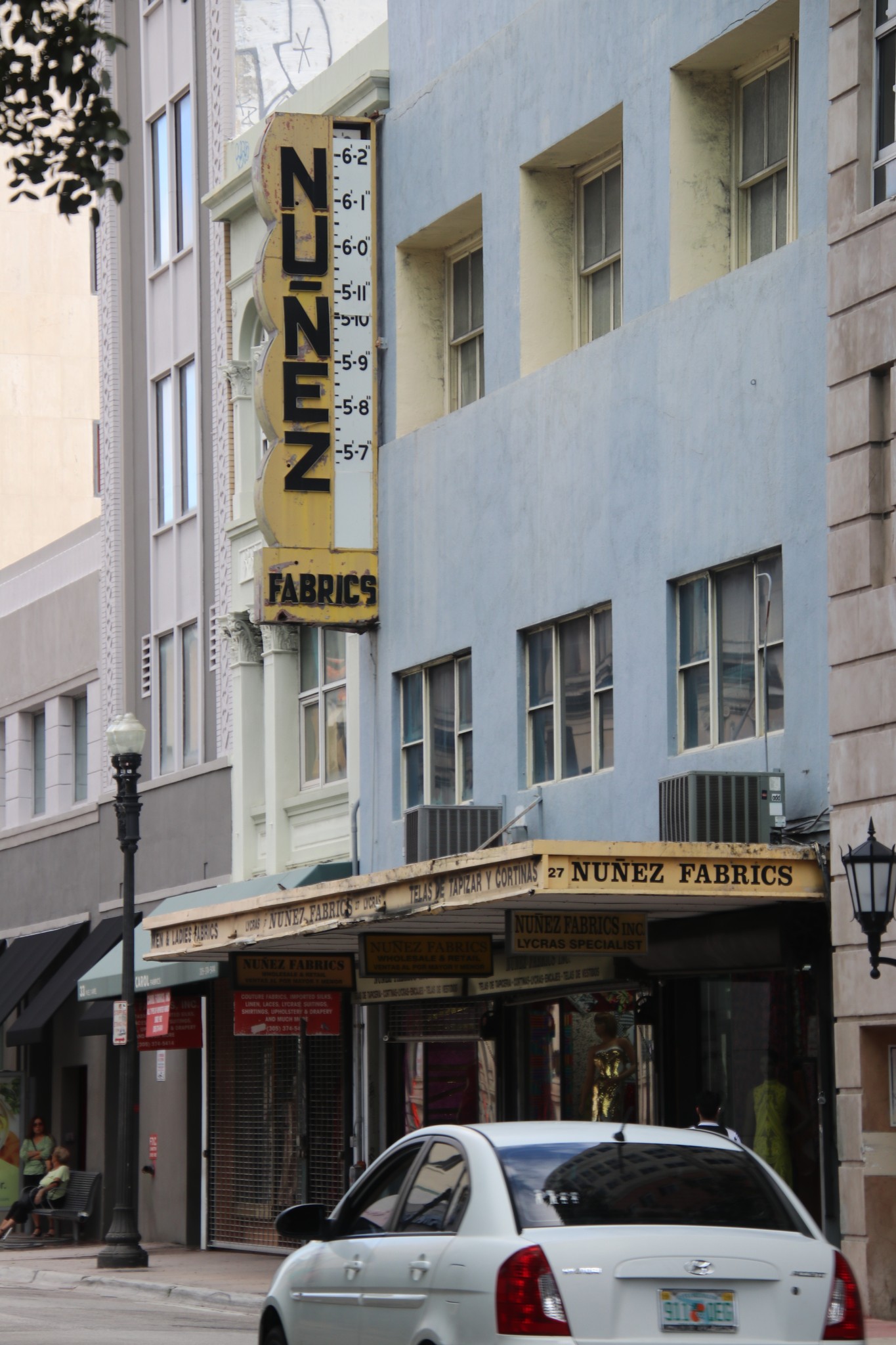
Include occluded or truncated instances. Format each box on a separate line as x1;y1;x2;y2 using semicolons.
95;711;150;1269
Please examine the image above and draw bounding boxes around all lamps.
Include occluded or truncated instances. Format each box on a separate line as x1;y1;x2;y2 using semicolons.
839;815;896;979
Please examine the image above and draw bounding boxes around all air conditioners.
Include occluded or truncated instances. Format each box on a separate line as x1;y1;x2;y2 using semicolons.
658;769;784;844
402;805;502;865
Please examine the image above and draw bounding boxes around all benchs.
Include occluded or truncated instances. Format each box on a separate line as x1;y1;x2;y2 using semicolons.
21;1171;101;1244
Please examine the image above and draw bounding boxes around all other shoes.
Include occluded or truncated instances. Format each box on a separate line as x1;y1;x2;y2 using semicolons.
0;1228;4;1239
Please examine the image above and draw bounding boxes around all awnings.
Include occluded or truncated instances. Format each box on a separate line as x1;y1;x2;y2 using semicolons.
0;911;148;1047
78;860;358;1001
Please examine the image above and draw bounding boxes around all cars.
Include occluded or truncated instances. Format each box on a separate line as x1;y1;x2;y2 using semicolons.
256;1118;870;1345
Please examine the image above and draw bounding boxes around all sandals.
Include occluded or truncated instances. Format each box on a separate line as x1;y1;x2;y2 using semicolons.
31;1226;43;1237
44;1227;56;1238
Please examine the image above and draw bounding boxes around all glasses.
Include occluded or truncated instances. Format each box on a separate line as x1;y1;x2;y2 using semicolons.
34;1122;43;1127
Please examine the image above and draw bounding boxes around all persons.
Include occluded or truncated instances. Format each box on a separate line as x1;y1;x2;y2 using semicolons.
19;1116;56;1238
0;1146;70;1237
